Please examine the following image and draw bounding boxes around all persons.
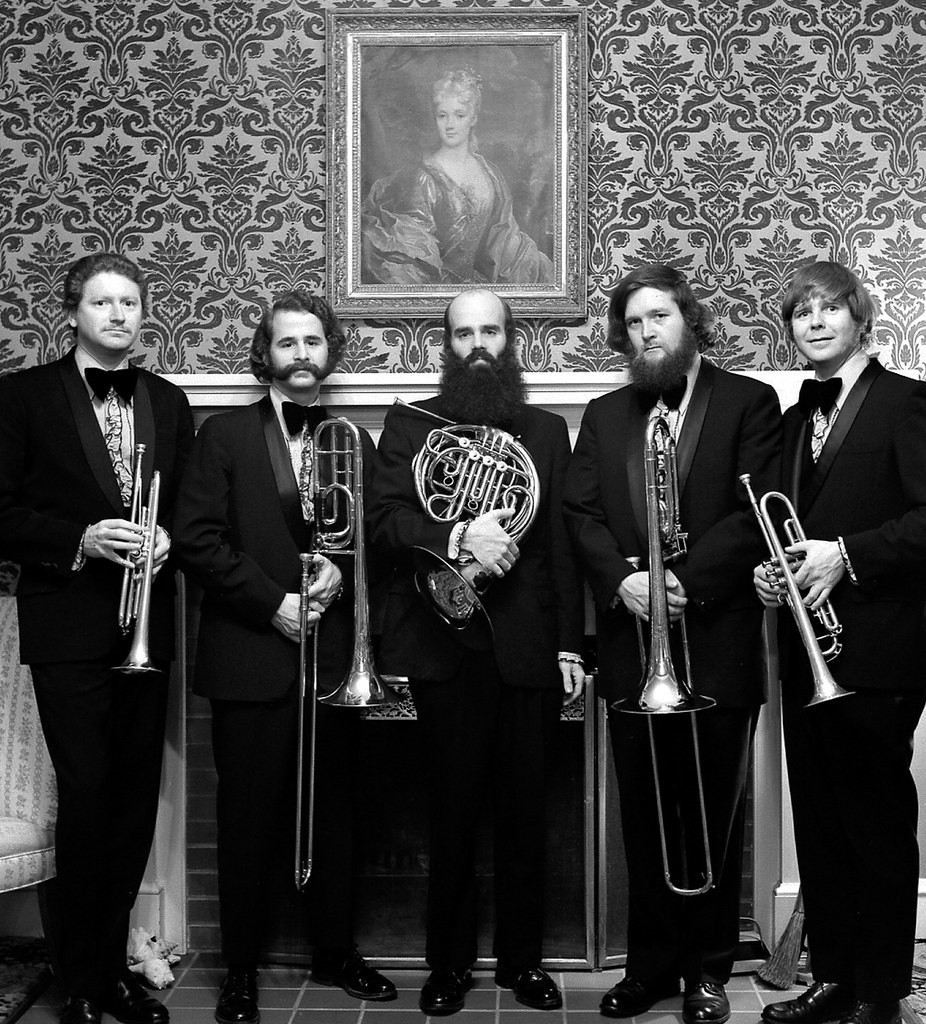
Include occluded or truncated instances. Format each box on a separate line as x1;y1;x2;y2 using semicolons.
779;262;926;1024
173;292;398;1024
372;288;573;1015
0;252;194;1024
563;265;782;1024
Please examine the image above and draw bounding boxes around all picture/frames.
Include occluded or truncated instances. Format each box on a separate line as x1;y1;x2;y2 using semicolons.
322;8;590;319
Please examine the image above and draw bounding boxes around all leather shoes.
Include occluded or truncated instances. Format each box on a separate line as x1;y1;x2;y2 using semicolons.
761;981;858;1024
61;990;100;1024
310;948;395;999
419;965;471;1011
840;998;899;1024
215;966;258;1024
99;967;170;1024
682;979;730;1024
494;957;562;1007
599;973;681;1018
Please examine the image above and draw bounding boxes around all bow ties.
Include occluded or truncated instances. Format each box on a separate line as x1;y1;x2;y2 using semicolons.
85;367;135;405
637;374;688;416
799;377;842;418
282;400;327;438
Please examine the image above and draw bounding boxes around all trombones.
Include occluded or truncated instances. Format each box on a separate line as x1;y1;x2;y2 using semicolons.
610;414;721;896
291;416;412;891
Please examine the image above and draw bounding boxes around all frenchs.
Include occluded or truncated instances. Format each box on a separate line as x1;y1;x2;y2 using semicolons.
391;396;542;646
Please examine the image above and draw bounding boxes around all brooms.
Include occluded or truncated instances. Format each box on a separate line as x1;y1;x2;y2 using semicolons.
755;884;806;991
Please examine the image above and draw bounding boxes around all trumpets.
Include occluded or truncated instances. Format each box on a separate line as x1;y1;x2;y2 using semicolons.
108;442;166;678
738;469;859;713
